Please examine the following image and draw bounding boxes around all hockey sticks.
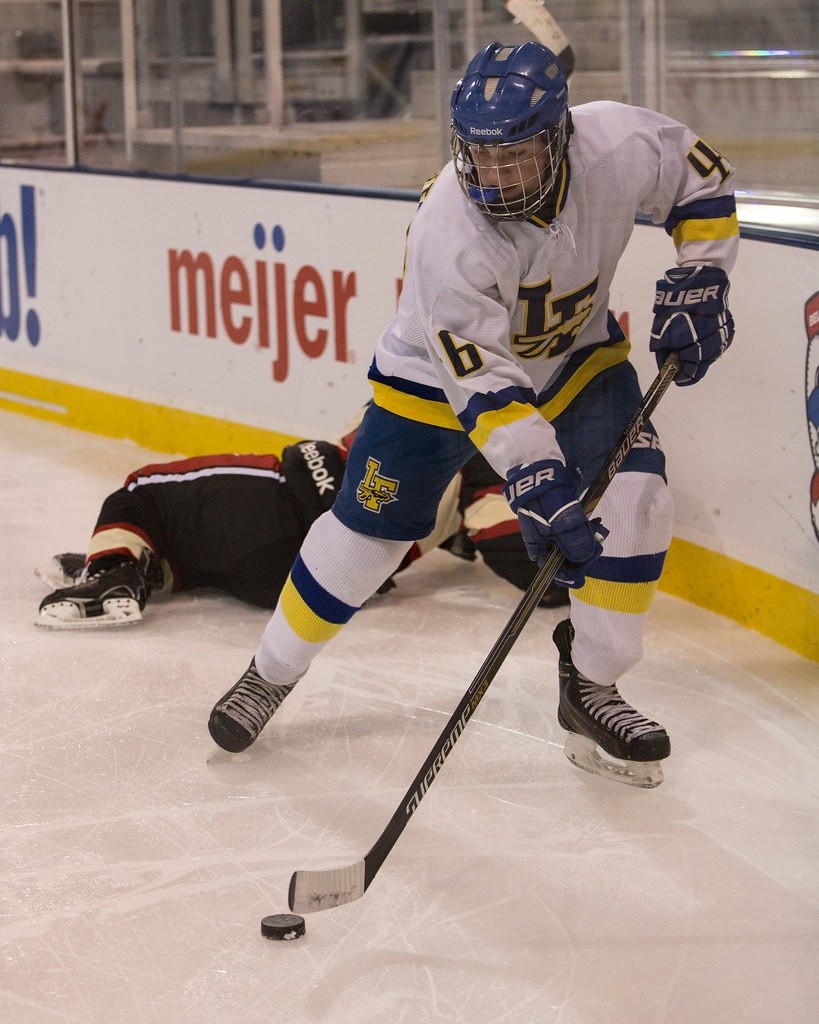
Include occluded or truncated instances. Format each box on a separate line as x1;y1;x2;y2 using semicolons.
286;352;681;917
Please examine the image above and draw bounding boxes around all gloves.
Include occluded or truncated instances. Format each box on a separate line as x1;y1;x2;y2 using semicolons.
502;459;611;588
650;265;736;387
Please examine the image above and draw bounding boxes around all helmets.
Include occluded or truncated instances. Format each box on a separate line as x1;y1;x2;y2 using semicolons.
450;40;575;222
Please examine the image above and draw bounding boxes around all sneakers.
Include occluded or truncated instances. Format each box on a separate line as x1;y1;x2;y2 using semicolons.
207;656;297;764
34;568;143;630
35;552;165;595
552;618;672;789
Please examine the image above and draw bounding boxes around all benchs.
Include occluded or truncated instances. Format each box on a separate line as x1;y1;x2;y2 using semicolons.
0;0;628;161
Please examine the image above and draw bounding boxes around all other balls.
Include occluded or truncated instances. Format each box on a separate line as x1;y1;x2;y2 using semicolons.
259;913;307;942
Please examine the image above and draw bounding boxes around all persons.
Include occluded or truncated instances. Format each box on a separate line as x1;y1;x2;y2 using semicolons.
34;421;569;630
209;41;741;788
